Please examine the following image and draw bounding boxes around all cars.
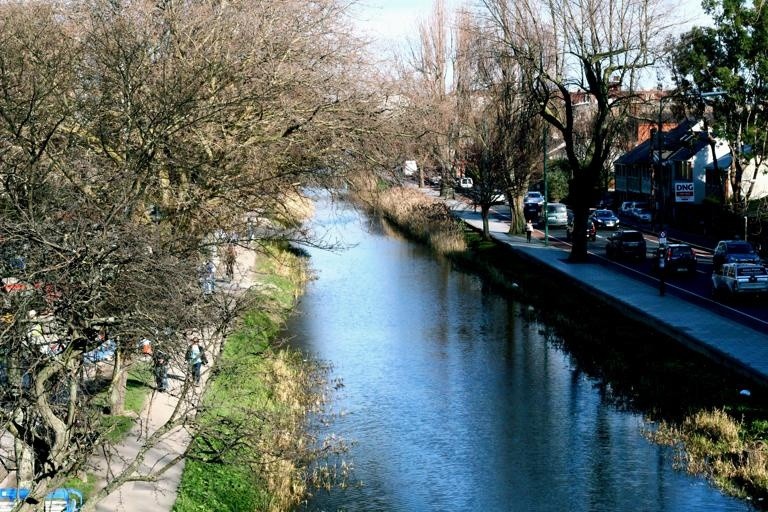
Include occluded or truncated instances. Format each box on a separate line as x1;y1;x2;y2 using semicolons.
402;157;621;244
607;198;767;303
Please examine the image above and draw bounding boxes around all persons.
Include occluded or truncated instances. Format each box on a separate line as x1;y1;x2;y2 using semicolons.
525;219;534;243
152;229;238;392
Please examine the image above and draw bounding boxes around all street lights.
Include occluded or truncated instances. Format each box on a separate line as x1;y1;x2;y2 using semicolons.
658;89;728;297
738;178;757;241
538;99;592;249
